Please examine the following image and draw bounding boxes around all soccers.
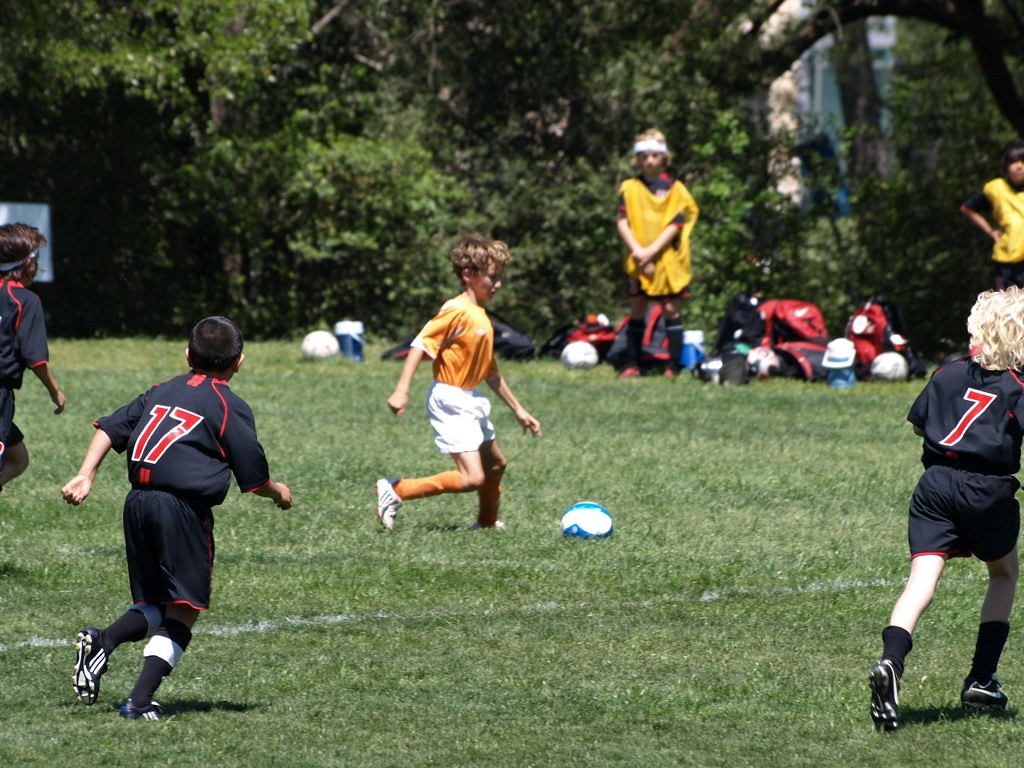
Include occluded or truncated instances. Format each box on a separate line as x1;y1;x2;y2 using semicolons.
560;501;614;540
301;330;341;360
870;351;908;383
746;345;781;379
560;340;598;370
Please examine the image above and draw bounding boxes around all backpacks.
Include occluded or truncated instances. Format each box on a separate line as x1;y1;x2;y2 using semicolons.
488;296;923;384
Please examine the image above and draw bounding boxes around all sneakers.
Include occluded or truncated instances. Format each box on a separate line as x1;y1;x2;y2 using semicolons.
868;659;899;732
375;478;403;532
72;627;107;707
120;699;167;722
961;674;1008;710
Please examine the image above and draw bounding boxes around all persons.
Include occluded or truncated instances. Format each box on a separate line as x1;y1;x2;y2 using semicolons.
376;235;541;533
0;222;64;492
616;128;699;379
61;316;291;721
869;286;1023;730
959;142;1024;289
